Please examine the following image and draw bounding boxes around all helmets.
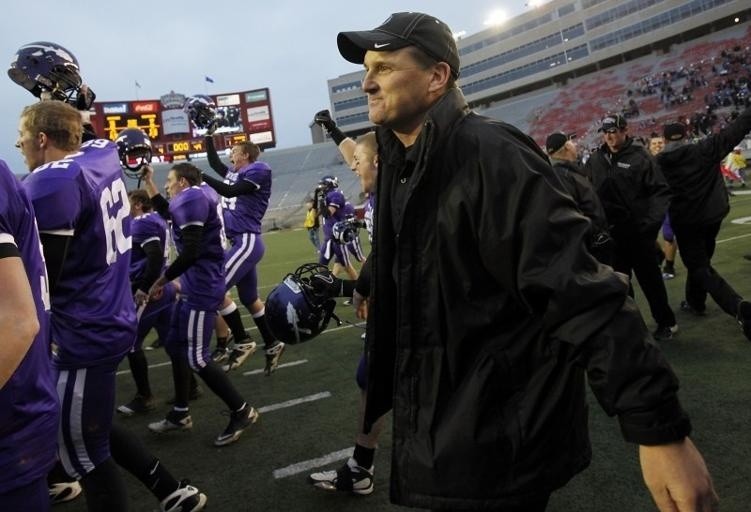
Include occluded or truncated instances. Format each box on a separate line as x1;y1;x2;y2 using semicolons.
184;96;216;130
332;216;363;244
114;127;152;177
319;175;339;189
264;273;337;344
8;41;81;103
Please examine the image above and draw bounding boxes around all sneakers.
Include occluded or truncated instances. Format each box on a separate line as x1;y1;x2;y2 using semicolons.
214;403;259;446
160;480;207;509
736;301;751;339
211;342;234;364
221;342;256;372
117;396;156;415
652;317;678;339
663;268;675;279
146;416;192;433
264;342;283;376
307;457;375;496
144;341;165;350
681;301;705;315
49;481;82;502
166;385;204;403
343;301;353;305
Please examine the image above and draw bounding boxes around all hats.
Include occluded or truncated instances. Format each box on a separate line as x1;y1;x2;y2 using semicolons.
546;132;578;153
664;122;686;141
598;115;626;133
337;12;460;75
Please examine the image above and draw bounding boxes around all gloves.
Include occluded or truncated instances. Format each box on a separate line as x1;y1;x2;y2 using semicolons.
207;121;218;135
314;110;336;137
307;271;342;299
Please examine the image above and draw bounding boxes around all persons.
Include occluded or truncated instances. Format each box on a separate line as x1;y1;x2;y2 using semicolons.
308;110;385;494
649;137;678;278
115;124;285;445
14;100;207;510
546;130;634;299
543;44;751;198
337;11;720;512
3;158;61;510
652;94;751;340
304;175;367;305
585;115;680;342
